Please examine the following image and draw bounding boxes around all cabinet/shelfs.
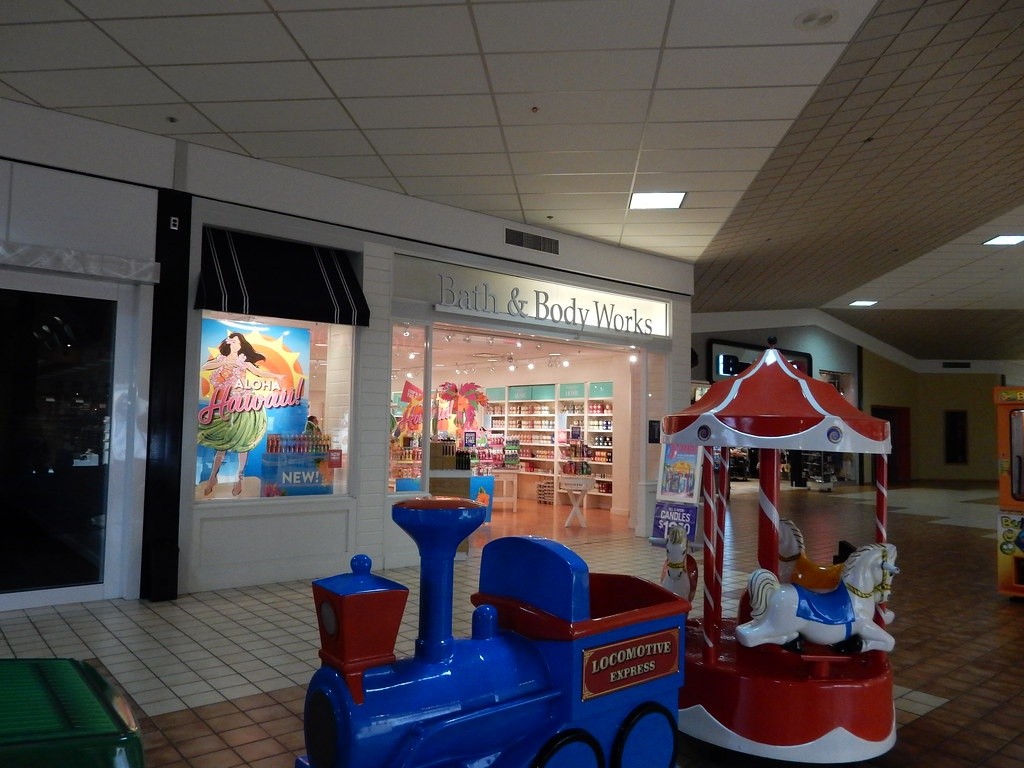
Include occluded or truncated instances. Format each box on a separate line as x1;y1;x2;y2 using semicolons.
388;436;422;488
482;397;612;510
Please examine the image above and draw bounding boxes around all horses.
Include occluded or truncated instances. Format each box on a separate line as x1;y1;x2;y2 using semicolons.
735;543;899;656
661;526;698;602
779;517;895;625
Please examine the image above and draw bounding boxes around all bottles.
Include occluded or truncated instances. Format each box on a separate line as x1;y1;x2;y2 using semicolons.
478;449;503;460
455;451;470;470
471;445;477;459
488;435;504;445
503;435;520;464
267;435;330;453
521;449;553;459
401;447;423;461
396;466;421;478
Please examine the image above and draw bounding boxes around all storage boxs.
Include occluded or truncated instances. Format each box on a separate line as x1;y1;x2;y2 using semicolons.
430;441;456;471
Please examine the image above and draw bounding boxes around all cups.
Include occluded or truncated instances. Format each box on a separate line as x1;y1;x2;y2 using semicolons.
472;465;493;476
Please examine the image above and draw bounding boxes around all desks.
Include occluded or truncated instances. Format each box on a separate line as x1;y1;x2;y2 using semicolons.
479;468;521;512
560;474;596;527
